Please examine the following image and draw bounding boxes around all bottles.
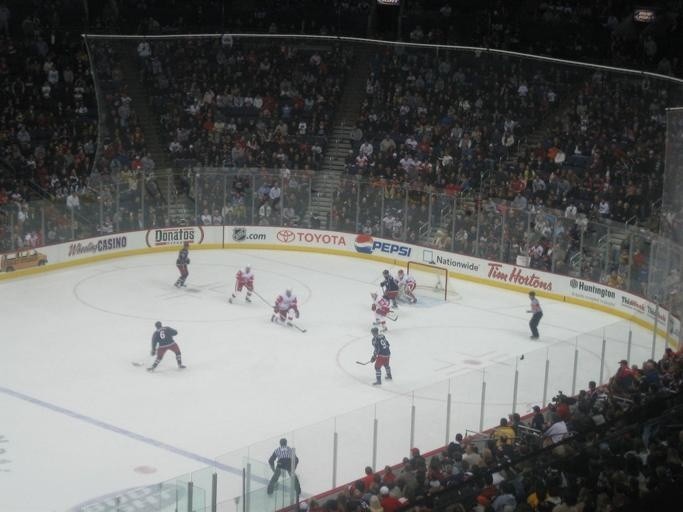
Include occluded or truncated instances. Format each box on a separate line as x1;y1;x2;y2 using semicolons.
618;360;627;363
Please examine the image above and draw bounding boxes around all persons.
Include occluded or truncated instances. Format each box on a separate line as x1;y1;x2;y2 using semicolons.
0;0;681;314
369;327;392;384
368;290;390;333
146;321;186;370
174;242;190;287
229;264;255;305
526;291;543;338
265;438;301;494
296;346;682;509
271;289;299;327
